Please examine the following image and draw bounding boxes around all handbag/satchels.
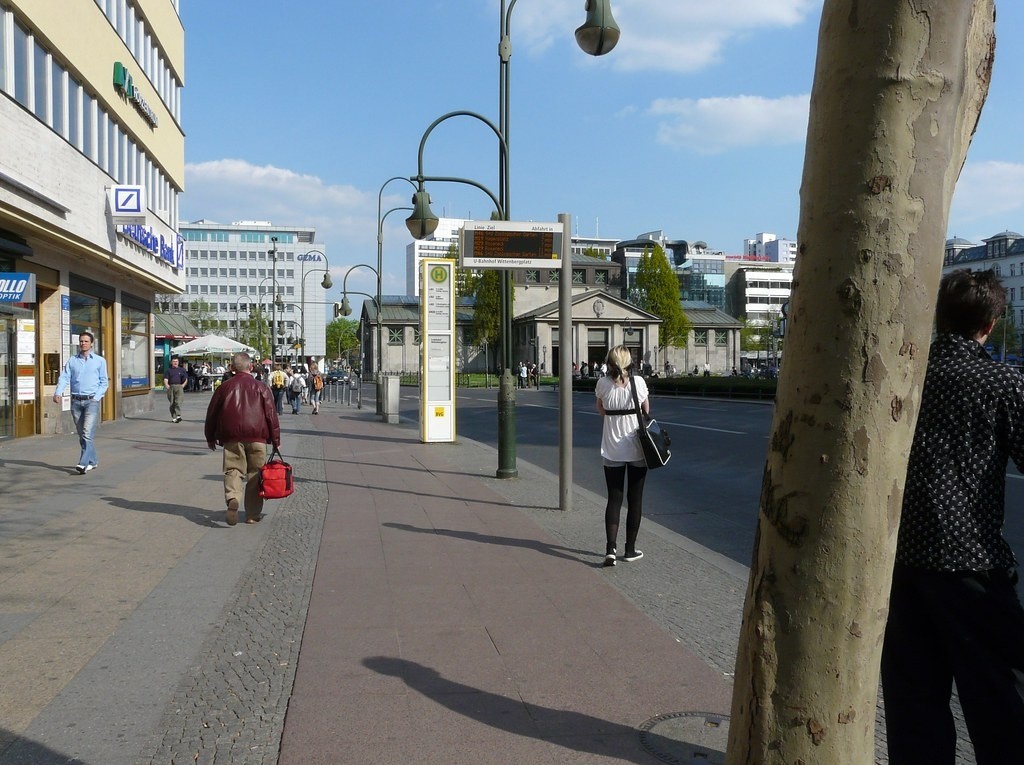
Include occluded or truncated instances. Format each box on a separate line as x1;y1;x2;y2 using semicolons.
259;447;294;500
639;418;672;470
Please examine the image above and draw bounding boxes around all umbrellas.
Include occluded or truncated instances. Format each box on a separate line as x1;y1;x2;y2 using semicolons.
170;334;255;373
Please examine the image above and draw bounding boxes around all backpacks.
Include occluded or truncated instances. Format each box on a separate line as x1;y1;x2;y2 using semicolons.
292;375;302;392
273;370;285;390
313;370;324;390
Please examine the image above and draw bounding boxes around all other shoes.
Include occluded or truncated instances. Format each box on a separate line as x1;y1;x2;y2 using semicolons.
312;407;318;415
624;550;643;562
292;409;299;415
605;548;617;567
177;416;182;421
76;464;86;474
87;465;97;470
226;499;239;526
245;517;259;523
172;418;178;423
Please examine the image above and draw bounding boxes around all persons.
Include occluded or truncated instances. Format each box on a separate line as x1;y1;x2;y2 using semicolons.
185;361;324;414
879;269;1024;765
205;352;280;527
594;345;649;565
517;359;737;389
53;333;108;474
163;358;187;423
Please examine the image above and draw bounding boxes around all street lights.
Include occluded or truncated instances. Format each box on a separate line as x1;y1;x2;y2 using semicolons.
278;249;334;373
406;0;622;484
234;277;283;375
337;176;418;416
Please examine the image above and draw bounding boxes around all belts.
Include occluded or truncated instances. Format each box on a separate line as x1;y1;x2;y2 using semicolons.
72;395;94;401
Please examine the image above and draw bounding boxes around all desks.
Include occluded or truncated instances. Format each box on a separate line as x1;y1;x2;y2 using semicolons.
201;374;223;392
